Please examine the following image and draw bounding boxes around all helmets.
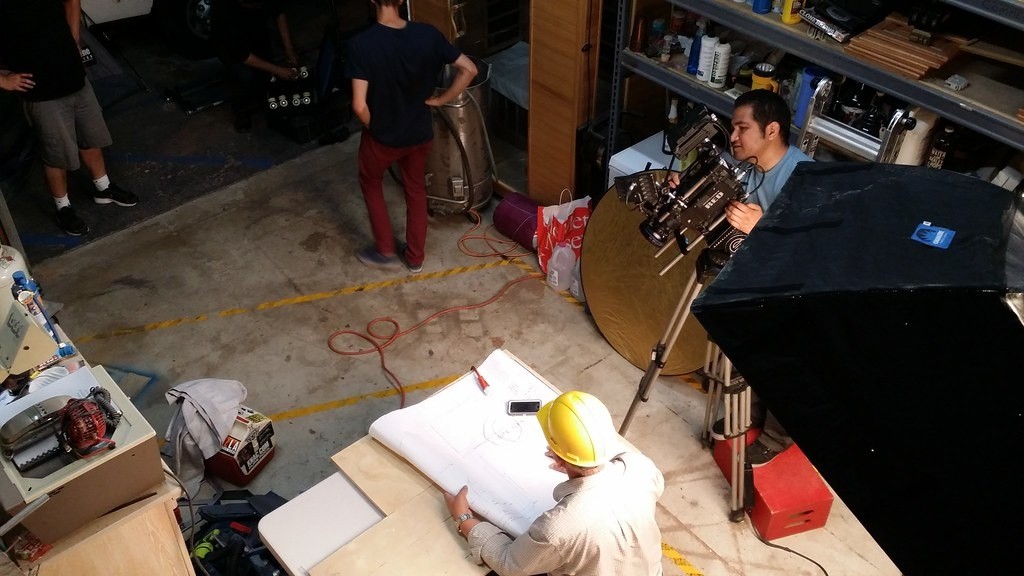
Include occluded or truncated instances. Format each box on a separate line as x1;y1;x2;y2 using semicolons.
536;388;616;467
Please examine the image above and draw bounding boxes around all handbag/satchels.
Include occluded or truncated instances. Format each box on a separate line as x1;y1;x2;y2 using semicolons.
537;188;590;275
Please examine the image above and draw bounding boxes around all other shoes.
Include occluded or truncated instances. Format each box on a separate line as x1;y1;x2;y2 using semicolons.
400;244;421;273
357;245;402;270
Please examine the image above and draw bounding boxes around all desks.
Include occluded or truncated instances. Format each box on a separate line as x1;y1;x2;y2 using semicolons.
578;170;742;375
258;469;387;576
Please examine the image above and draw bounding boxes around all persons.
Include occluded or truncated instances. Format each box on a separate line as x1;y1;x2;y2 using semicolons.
347;0;478;273
0;0;138;235
660;90;816;467
210;0;301;84
444;391;664;576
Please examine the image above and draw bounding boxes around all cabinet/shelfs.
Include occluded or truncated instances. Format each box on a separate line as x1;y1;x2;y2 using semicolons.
612;0;1024;287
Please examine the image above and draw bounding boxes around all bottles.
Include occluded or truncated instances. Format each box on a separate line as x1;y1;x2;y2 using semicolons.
732;0;807;25
854;90;888;136
546;241;576;293
630;10;732;89
794;66;826;129
569;255;586;303
665;97;679;126
12;270;55;337
924;125;954;169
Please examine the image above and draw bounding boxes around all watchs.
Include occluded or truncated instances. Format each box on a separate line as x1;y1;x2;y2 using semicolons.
454;514;474;532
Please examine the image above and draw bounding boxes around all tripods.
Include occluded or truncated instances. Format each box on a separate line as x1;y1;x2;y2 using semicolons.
619;225;753;523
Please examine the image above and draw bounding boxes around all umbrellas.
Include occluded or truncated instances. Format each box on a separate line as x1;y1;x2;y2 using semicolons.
689;160;1024;576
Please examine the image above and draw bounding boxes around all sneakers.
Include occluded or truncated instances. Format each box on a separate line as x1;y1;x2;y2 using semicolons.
711;414;732;439
95;183;137;207
741;442;777;471
55;207;90;236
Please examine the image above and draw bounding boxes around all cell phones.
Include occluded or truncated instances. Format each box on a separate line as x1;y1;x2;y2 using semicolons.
508;400;541;416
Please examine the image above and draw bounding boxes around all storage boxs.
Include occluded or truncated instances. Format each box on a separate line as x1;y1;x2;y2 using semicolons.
715;429;833;541
608;130;691;190
5;364;164;546
204;404;275;489
